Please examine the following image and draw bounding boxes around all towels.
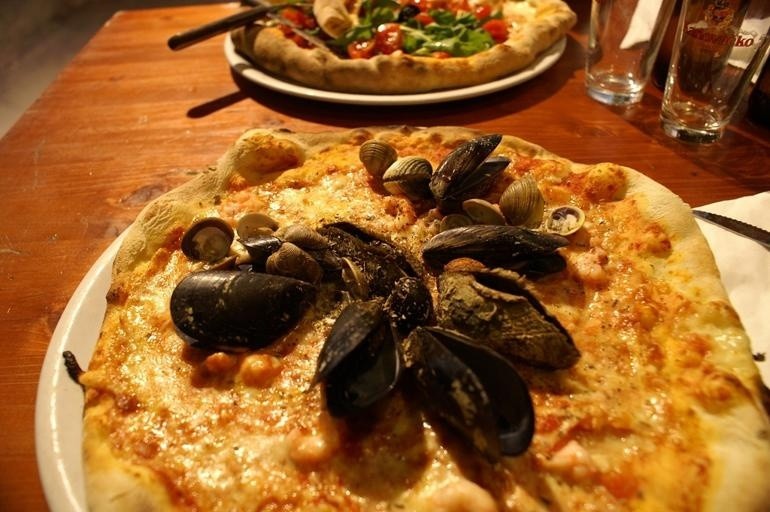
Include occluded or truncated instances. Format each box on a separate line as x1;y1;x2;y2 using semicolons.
691;190;769;386
619;1;770;85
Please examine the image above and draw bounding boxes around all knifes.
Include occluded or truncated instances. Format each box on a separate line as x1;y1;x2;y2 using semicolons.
694;210;769;249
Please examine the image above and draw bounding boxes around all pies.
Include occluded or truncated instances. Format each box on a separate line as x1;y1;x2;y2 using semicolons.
231;0;579;94
81;125;770;512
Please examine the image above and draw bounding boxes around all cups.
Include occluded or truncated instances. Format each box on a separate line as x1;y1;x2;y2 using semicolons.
585;1;768;148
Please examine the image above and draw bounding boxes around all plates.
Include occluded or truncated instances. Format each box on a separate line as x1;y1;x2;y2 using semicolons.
221;29;569;107
32;222;138;512
